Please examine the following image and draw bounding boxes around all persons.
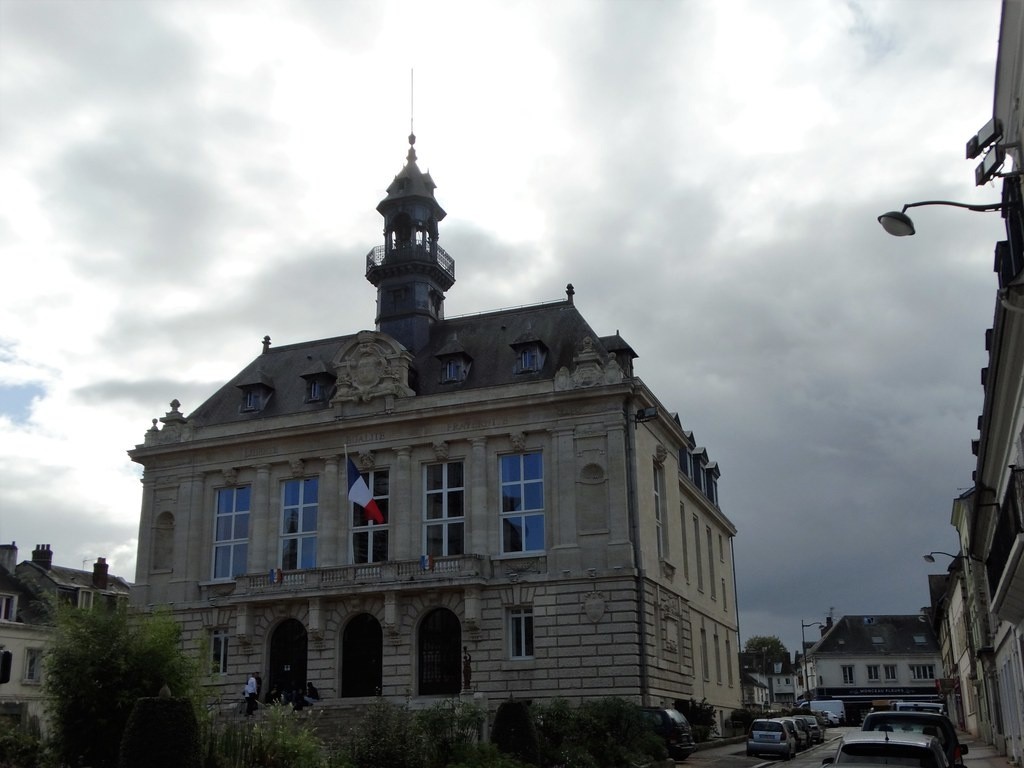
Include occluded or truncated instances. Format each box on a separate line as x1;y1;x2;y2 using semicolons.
303;682;320;704
244;671;262;717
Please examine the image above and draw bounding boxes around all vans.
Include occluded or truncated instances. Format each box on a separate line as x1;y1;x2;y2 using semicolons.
797;699;847;726
893;701;946;714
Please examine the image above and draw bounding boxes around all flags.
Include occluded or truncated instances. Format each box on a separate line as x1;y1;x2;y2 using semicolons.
348;455;385;525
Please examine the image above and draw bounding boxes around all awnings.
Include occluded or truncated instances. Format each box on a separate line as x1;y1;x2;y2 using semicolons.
935;678;955;688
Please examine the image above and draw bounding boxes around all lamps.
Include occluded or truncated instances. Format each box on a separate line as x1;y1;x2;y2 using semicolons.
878;201;1018;238
917;615;946;624
921;552;970;566
630;405;660;430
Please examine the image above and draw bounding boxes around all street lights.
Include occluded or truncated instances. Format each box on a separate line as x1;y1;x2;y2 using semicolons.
802;620;825;702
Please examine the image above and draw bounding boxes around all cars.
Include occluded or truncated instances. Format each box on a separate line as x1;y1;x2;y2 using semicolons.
772;709;840;752
821;729;969;768
746;719;796;761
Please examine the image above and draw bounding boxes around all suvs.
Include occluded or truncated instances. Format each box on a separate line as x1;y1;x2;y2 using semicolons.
638;705;695;762
861;707;969;768
